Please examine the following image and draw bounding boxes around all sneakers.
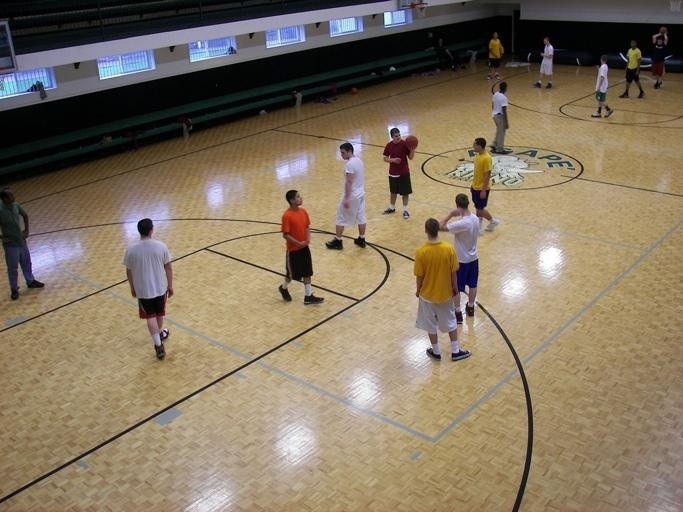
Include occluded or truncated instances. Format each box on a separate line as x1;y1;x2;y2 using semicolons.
486;72;501;81
278;284;293;303
590;76;665;118
353;235;367;248
451;348;473;362
382;205;397;216
426;347;442;361
325;236;344;250
485;218;499;231
453;308;464;324
154;327;169;359
465;302;476;317
533;81;553;89
10;280;46;301
402;209;410;220
303;292;324;305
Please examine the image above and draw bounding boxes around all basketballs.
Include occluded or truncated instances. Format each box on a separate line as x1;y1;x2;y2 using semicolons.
405;135;418;148
350;88;359;94
659;27;667;34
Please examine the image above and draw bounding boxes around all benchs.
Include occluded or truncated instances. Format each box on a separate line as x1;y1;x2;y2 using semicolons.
0;42;469;183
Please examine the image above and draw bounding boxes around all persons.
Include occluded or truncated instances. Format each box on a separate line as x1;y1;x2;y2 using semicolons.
439;194;480;322
470;138;499;234
424;38;465;70
533;37;554;88
487;32;503;80
413;219;472;360
652;32;669;89
491;82;508;154
0;187;44;299
123;219;173;359
325;143;366;249
382;128;416;219
619;40;645;98
278;190;323;304
591;55;613;117
